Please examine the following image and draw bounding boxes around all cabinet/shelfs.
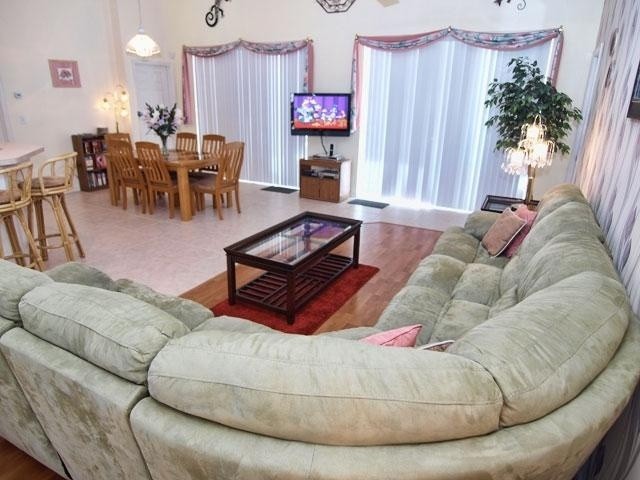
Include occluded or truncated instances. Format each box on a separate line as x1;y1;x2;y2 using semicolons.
300;157;350;205
70;132;112;194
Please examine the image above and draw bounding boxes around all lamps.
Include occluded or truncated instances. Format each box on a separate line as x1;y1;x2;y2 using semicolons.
99;83;131;133
499;111;557;215
123;0;161;58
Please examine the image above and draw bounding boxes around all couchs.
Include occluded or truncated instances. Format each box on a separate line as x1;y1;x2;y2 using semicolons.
0;184;638;480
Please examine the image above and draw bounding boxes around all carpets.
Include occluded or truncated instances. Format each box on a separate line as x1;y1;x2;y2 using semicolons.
261;180;299;195
346;197;389;212
200;253;380;334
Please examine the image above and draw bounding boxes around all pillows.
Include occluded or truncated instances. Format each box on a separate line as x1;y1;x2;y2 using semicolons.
362;322;461;359
484;201;534;260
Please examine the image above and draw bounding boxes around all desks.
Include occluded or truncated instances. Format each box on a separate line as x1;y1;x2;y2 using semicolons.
481;191;540;216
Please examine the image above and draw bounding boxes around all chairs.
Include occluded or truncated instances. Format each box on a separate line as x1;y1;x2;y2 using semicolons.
102;127;246;223
1;150;87;273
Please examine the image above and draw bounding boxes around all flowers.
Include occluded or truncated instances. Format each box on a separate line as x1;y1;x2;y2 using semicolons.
138;103;188;140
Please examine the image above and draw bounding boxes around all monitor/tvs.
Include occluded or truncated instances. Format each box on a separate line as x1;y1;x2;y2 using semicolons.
290;92;351;137
